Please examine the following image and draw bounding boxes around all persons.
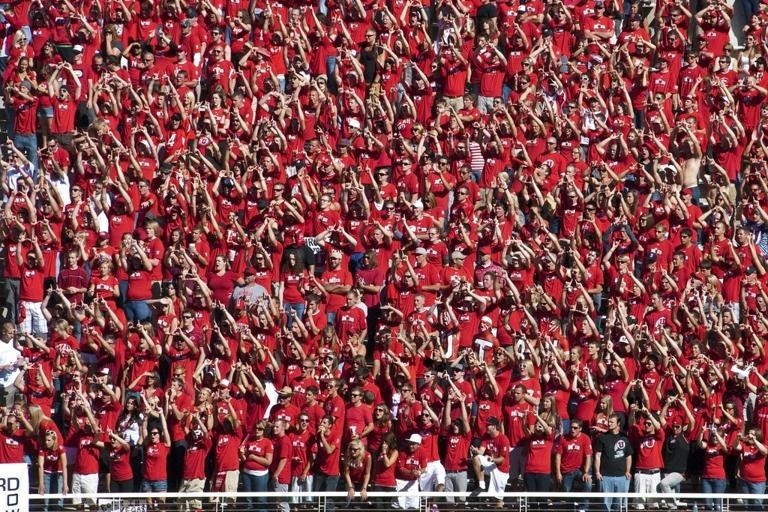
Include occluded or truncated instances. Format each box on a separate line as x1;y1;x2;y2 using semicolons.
1;1;768;512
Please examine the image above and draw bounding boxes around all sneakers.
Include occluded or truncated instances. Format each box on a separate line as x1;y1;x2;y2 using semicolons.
472;486;486;494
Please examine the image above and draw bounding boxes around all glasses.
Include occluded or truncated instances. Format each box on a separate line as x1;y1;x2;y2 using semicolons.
5;1;732;452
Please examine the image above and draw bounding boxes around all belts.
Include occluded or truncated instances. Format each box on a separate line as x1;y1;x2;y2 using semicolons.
447;469;467;473
635;469;660;474
